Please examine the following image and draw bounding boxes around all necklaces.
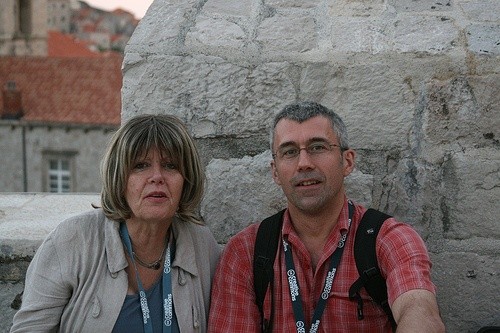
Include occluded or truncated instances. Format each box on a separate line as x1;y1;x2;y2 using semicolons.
130;250;166;271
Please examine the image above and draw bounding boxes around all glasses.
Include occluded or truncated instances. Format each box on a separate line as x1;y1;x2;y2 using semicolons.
272;143;344;161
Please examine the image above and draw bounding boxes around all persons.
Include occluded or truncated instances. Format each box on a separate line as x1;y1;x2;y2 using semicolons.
206;100;445;333
9;113;221;333
